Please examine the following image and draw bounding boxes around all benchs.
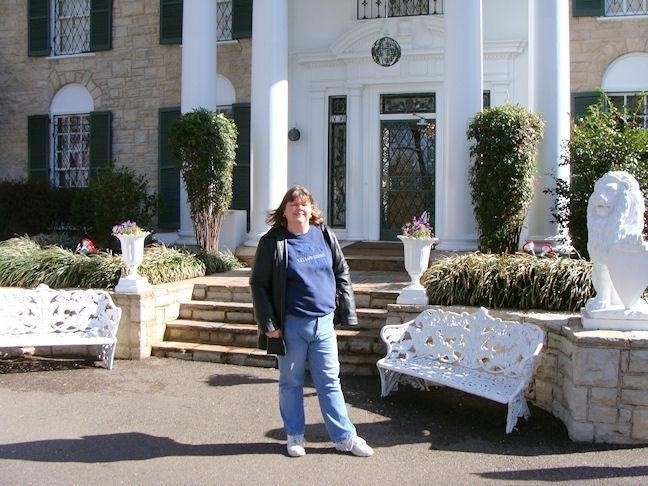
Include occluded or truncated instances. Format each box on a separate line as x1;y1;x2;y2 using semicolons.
375;305;544;435
0;283;122;370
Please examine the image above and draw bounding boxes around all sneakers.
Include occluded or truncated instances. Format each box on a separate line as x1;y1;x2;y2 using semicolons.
335;436;374;457
287;437;306;456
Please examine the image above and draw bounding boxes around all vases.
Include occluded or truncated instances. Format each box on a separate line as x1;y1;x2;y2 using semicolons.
395;234;440;281
114;231;152;271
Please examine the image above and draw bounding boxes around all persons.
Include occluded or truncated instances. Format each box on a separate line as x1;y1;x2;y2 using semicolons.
249;185;374;457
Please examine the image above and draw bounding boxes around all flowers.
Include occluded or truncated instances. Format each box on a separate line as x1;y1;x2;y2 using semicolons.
401;210;432;238
112;219;145;234
